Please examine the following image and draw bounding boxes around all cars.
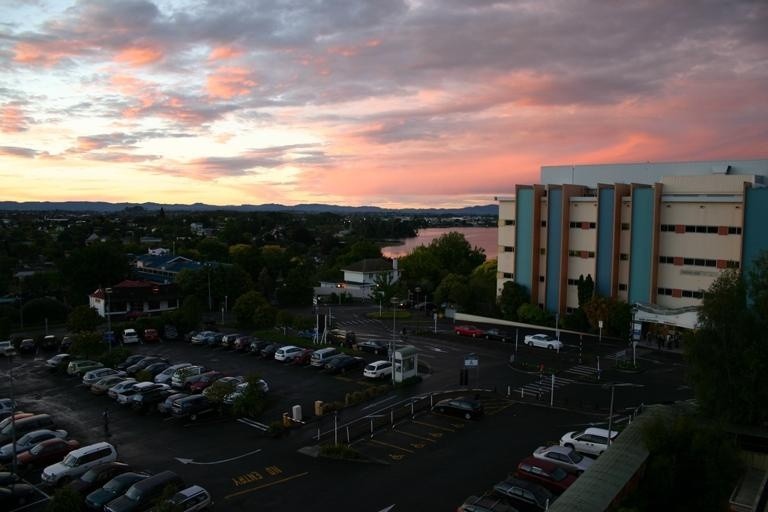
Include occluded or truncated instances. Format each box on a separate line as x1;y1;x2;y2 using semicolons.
457;443;595;512
523;333;565;350
454;324;513;342
399;299;436;309
558;426;619;457
431;396;483;420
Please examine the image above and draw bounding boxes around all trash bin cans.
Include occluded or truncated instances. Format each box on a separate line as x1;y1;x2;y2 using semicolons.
292;400;323;420
460;368;468;386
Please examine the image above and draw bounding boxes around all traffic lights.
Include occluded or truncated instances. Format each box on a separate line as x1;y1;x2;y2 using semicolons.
336;284;344;288
